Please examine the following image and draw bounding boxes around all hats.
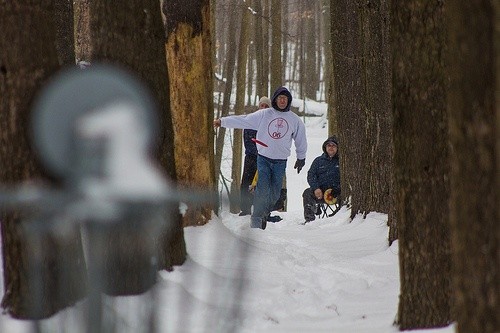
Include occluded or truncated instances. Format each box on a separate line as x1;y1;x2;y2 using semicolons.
259;96;270;107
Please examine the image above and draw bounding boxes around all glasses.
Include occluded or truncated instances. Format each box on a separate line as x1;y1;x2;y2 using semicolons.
328;144;335;147
278;97;287;100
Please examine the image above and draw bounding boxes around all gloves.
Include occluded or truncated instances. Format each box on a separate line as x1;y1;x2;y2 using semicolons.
294;159;305;174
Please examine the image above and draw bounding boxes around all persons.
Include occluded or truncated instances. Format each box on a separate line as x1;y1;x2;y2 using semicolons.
302;137;348;225
213;86;308;230
238;96;284;223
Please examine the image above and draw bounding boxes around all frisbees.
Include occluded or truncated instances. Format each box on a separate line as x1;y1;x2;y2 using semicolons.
251;138;268;147
324;189;337;205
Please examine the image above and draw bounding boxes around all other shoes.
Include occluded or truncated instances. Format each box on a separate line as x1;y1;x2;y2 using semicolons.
239;210;251;216
267;215;281;222
305;216;315;223
262;218;266;229
